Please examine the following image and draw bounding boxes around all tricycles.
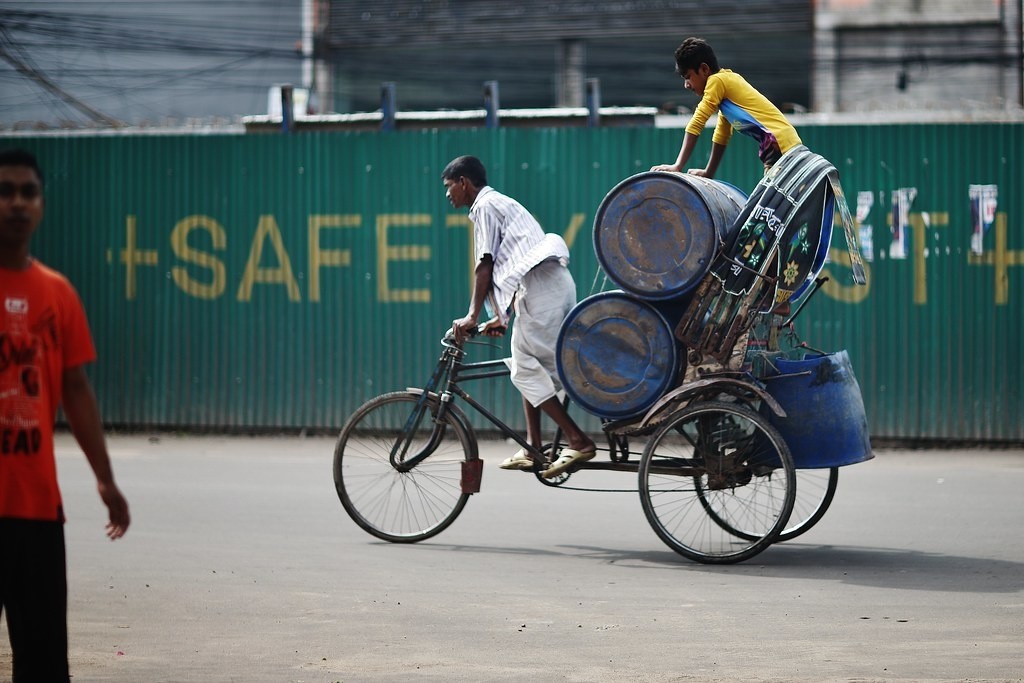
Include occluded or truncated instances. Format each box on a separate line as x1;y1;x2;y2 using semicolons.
333;143;866;566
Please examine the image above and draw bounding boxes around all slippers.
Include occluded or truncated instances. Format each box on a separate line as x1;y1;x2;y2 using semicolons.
498;449;548;469
541;448;595;477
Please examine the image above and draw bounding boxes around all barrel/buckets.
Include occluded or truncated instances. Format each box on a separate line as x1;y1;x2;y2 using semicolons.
554;290;687;420
591;170;749;301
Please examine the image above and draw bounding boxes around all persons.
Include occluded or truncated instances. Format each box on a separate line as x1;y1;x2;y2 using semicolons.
0;154;131;683
441;155;597;480
650;36;803;317
